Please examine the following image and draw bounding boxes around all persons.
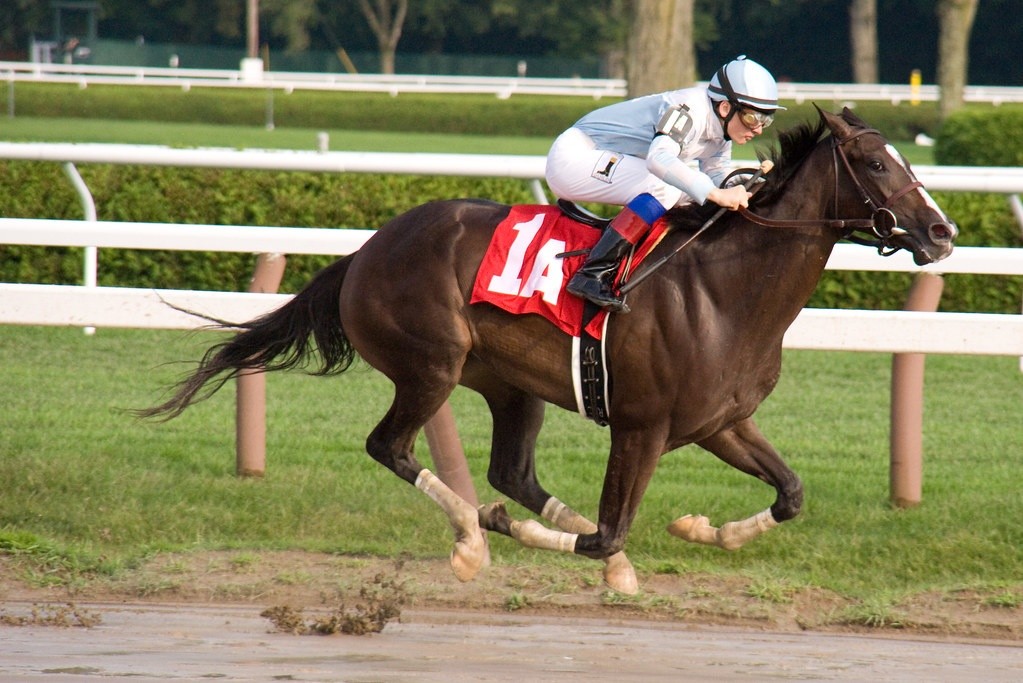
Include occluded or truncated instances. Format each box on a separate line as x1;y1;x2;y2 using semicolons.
545;54;790;313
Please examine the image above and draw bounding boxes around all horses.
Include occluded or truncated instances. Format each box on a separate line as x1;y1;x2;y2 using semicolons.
130;102;961;596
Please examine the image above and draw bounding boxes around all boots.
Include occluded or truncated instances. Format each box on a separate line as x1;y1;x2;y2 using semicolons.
565;193;666;312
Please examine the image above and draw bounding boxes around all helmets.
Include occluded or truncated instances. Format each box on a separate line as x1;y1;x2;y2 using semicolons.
707;55;788;111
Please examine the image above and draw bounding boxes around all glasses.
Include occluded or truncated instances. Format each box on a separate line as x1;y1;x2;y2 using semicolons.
741;112;774;128
